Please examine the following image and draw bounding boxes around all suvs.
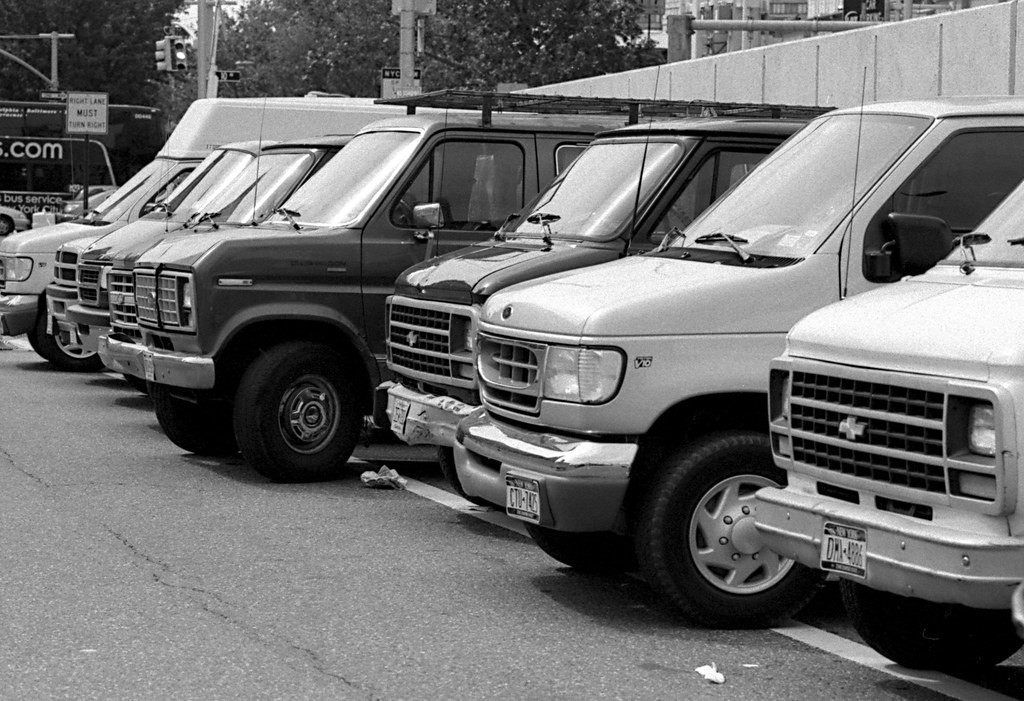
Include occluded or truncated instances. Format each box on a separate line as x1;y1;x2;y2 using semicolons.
746;179;1024;670
0;90;835;483
373;114;805;460
443;96;1024;630
